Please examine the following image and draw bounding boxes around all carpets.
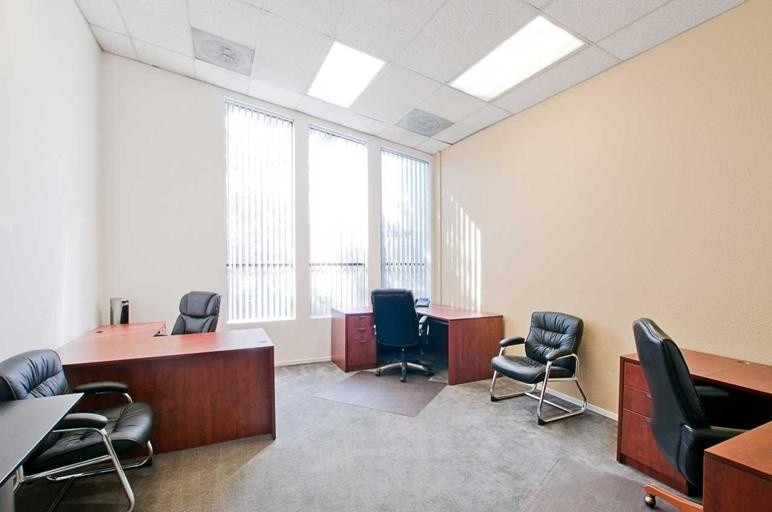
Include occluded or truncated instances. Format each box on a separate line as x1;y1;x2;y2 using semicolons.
520;455;682;512
313;371;447;417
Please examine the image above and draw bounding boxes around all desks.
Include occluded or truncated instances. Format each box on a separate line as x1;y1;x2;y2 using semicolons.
616;347;772;512
53;321;276;464
331;303;504;386
0;392;85;489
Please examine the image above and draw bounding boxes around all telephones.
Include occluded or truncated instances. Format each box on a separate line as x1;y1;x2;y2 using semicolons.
415;297;430;307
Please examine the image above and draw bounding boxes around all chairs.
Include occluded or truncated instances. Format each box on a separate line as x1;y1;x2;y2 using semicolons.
489;311;587;424
0;348;155;512
171;291;221;335
371;288;435;383
632;318;749;512
